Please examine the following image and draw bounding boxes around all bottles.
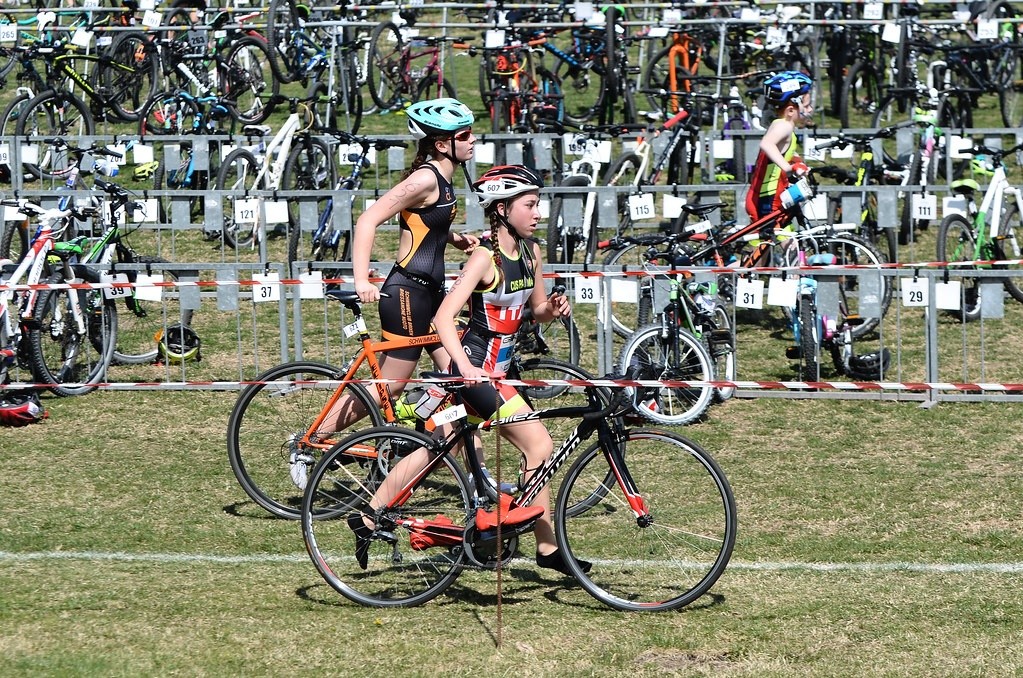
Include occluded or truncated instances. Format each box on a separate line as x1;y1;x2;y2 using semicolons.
414;368;449;418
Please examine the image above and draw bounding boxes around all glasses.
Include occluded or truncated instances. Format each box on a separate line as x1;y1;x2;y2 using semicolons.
445;126;471;142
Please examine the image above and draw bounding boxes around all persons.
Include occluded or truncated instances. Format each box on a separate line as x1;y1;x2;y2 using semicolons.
727;70;814;268
284;97;518;503
166;11;213;49
344;164;592;575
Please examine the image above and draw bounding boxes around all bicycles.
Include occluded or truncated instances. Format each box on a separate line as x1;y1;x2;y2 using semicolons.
226;285;628;523
299;361;740;613
0;0;1023;430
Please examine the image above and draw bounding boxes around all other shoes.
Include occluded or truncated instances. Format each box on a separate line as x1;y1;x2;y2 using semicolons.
465;472;519;502
286;433;308;491
537;547;591;575
349;512;374;569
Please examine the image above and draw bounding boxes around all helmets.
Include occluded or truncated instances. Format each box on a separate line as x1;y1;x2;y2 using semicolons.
850;348;891;379
763;71;813;103
0;389;42;425
715;161;733;181
160;323;199;364
404;98;474;139
472;165;543;208
